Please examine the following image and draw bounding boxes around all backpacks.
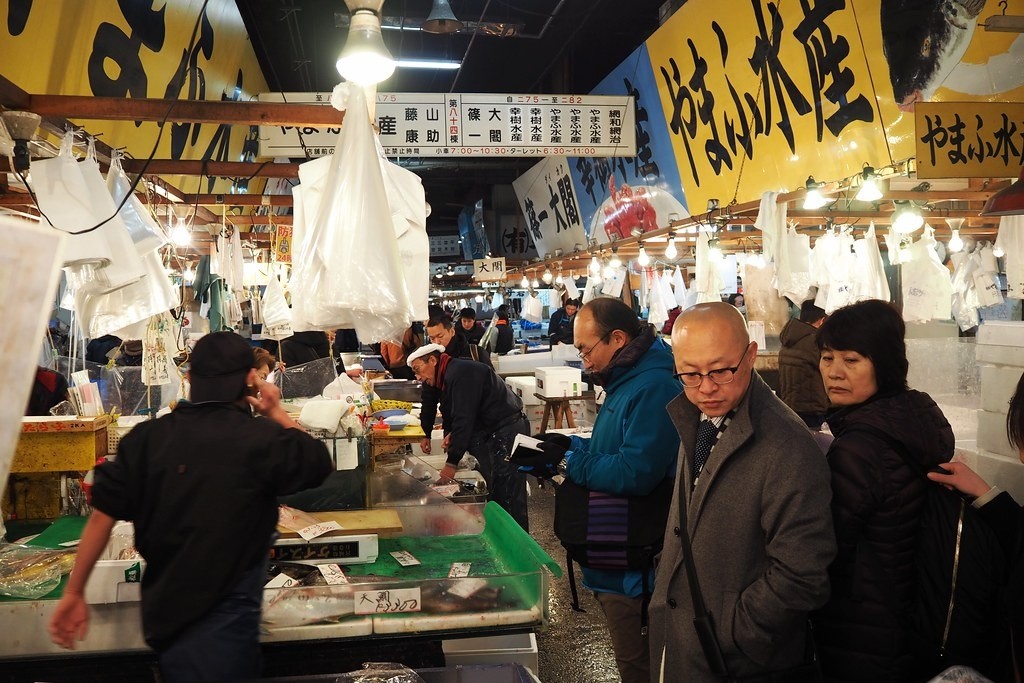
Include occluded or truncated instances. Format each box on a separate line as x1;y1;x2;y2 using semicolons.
836;426;1015;674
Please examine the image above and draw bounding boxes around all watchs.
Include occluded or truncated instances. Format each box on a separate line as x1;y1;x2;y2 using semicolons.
556;458;568;479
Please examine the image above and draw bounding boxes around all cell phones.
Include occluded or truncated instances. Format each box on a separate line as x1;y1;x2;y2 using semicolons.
248;377;257;400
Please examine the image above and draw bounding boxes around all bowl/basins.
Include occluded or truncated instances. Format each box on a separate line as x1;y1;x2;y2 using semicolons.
384;421;408;430
372;409;407;420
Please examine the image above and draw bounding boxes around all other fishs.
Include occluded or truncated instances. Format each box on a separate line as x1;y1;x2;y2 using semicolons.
268;562;501;612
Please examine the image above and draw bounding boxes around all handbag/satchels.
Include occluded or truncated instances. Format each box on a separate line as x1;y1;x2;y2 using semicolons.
553;470;678;572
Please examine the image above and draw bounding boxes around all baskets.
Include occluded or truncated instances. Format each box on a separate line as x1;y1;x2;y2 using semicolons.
372;400;412;414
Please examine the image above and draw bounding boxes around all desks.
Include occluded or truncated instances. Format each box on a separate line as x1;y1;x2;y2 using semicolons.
369;424;426;472
533;390;595;434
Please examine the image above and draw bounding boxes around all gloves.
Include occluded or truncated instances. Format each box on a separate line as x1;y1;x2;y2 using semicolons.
516;433;572;478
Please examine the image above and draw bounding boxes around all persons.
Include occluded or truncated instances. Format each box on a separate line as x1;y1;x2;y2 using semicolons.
660;305;682;334
728;294;745;321
103;338;163;416
266;322;426;380
548;298;584;352
812;299;961;683
407;342;531;537
777;298;830;434
649;301;837;683
381;302;513;380
927;371;1024;683
420;314;497;455
531;298;686;683
47;331;343;683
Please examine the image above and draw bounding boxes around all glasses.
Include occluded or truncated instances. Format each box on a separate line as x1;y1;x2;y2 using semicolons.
413;357;429;374
672;344;749;388
579;328;625;363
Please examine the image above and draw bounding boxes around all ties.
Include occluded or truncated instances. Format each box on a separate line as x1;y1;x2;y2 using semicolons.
690;420;718;491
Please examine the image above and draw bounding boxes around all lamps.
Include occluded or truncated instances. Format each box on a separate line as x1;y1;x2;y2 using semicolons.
445;263;474;276
421;0;465;35
2;110;43;174
496;268;518;294
856;166;882;203
551;283;567;297
435;266;448;278
335;0;396;84
944;217;965;252
169;203;192;246
803;177;827;211
889;198;923;234
526;286;539;298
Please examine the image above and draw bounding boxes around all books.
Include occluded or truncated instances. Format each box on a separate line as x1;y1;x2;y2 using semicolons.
509;433;545;468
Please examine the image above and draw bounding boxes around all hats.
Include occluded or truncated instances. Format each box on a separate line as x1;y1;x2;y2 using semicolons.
189;332;256;404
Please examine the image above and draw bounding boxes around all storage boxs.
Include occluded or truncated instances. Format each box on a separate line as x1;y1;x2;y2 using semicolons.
498;344;587;373
516;379;542;406
9;415;110;473
266;662;543;683
950;318;1024;508
84;559;146;605
505;375;535;392
402;429;489;505
442;632;534;676
0;472;61;521
0;597;153;659
534;366;583;398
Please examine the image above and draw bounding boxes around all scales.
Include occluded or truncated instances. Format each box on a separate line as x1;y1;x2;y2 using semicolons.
360;355;386;372
